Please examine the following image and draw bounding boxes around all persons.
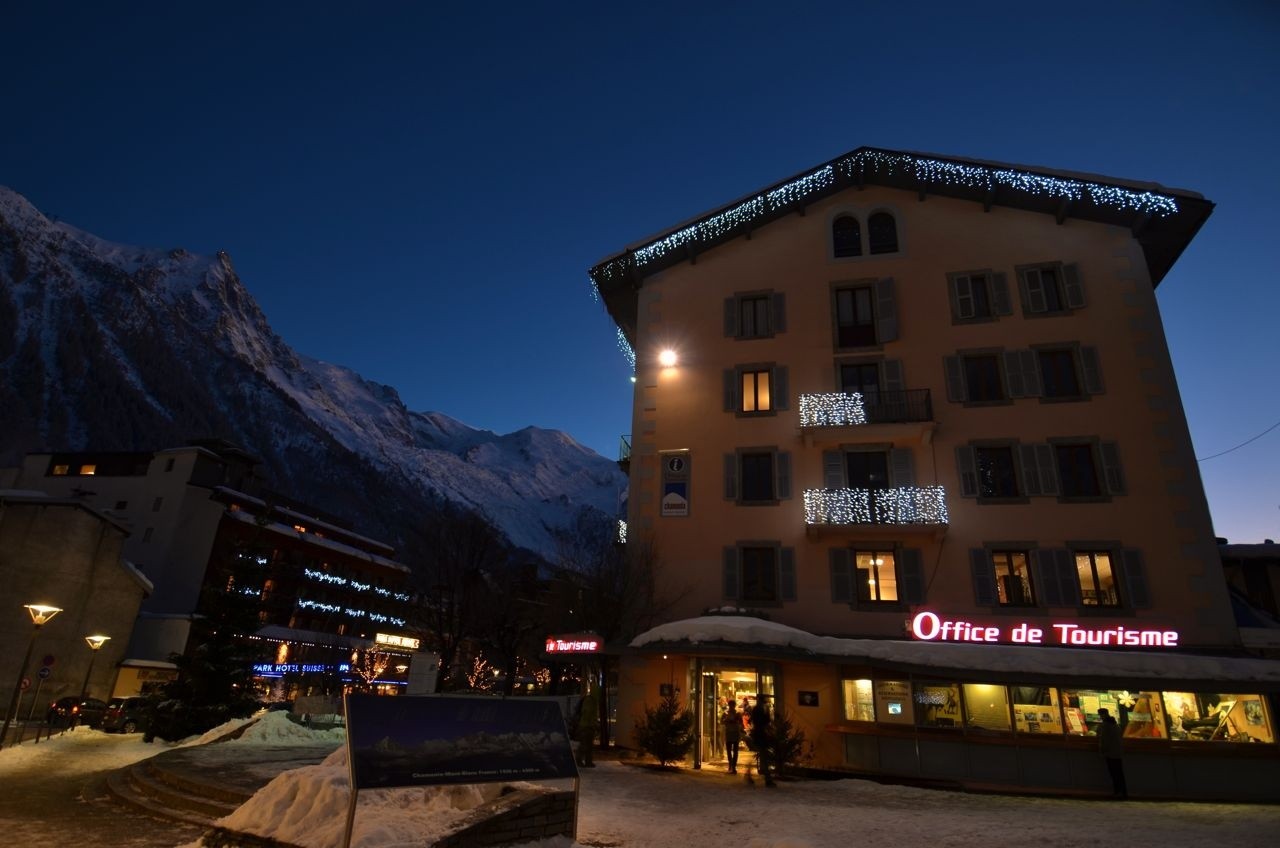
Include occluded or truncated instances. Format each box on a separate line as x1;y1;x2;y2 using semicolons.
1097;708;1128;800
720;701;744;774
576;688;600;767
742;697;753;751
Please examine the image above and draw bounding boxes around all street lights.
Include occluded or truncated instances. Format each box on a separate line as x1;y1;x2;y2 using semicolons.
1;605;66;747
71;635;110;732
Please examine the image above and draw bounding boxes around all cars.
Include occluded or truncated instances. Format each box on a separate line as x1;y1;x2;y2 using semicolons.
103;695;168;734
46;697;105;728
